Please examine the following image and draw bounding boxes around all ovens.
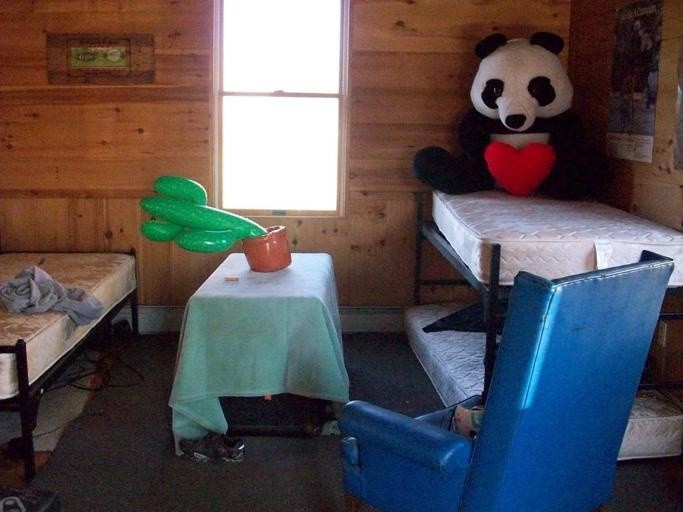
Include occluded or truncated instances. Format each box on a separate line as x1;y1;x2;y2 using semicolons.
0;247;139;483
403;187;683;462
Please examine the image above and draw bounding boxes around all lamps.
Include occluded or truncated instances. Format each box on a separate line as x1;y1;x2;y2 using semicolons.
337;248;676;512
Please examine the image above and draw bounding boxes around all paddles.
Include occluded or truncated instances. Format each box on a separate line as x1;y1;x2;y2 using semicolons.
24;332;681;512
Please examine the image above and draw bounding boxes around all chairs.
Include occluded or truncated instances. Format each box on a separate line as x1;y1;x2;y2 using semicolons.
181;432;245;464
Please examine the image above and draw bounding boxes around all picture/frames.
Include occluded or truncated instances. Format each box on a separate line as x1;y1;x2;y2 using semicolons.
168;252;352;456
